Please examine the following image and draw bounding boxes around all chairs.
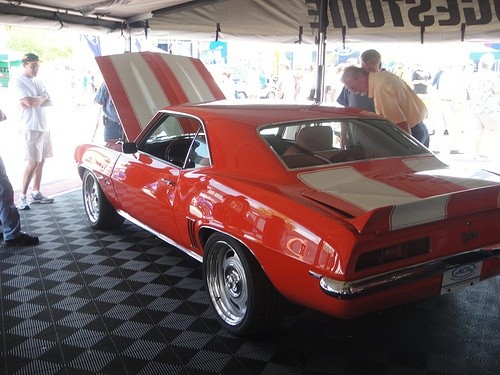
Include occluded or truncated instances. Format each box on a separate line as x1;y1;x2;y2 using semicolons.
283;126;338;154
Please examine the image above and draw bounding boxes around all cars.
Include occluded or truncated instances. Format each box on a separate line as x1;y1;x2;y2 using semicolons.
75;51;500;333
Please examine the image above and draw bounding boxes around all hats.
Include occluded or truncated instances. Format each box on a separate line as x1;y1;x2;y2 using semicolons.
22;53;43;64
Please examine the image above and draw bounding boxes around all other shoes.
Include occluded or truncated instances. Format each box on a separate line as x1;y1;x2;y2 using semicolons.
5;233;39;247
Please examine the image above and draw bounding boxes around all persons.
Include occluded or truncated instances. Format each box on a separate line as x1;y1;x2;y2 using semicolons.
94;82;123;142
16;53;56;210
340;65;430;149
411;58;432;136
277;57;316;100
431;53;500;159
223;70;245;98
244;66;261;101
334;48;382;149
0;109;39;247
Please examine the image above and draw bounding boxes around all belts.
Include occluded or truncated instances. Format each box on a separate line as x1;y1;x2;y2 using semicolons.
107;120;120;126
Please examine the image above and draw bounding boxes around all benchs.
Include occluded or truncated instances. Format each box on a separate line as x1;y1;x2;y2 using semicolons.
280;145;391;169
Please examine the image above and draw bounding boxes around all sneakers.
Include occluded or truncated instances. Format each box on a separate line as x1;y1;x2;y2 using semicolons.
30;191;55;204
15;197;31;210
